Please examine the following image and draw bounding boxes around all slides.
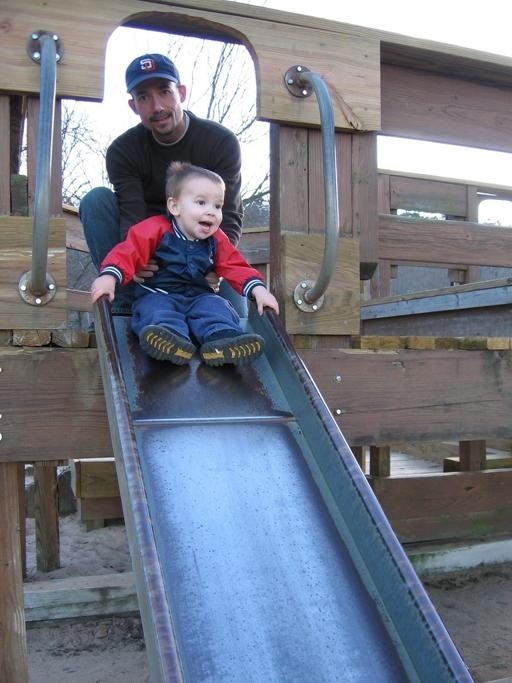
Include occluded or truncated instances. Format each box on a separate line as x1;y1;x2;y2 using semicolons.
93;294;476;683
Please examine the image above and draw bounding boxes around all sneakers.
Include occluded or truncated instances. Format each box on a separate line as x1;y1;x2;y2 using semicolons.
110;296;134;314
139;326;196;366
200;332;266;367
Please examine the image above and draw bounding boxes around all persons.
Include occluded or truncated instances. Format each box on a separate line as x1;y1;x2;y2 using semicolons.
75;52;251;320
87;158;282;368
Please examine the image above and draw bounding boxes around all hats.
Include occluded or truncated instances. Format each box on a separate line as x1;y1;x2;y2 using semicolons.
125;54;179;93
